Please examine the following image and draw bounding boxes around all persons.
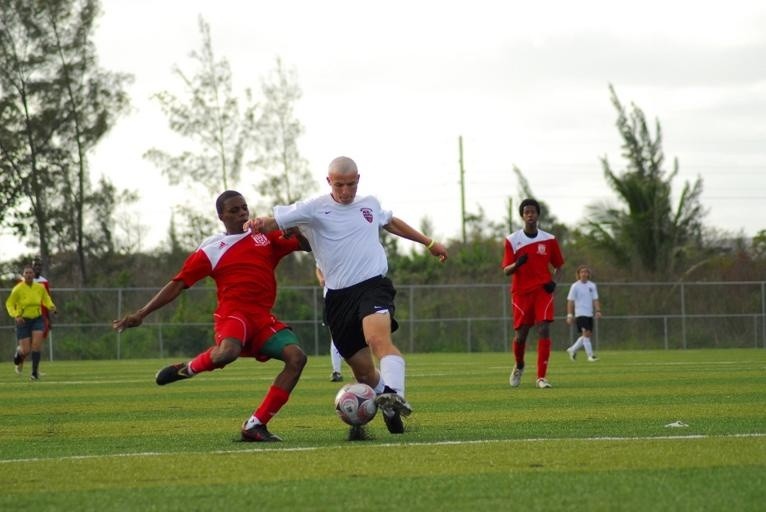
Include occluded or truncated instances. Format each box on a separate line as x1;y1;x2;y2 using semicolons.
500;198;565;390
566;263;602;362
19;256;51;377
316;261;352;382
112;189;312;443
241;155;449;433
4;264;57;379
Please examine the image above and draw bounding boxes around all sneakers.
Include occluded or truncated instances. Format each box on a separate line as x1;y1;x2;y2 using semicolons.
329;370;343;383
587;353;600;361
14;362;23;375
376;392;412;416
155;362;189;385
536;376;551;389
509;361;525;387
566;347;576;362
241;420;284;442
381;385;403;433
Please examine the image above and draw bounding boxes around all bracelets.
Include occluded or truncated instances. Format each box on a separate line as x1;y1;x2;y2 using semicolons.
596;310;601;312
427;239;435;249
567;313;573;318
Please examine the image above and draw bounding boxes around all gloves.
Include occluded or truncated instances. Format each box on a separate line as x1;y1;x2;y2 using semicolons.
544;281;556;292
519;253;528;265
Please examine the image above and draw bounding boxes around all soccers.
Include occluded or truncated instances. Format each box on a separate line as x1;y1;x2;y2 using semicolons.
334;383;376;426
